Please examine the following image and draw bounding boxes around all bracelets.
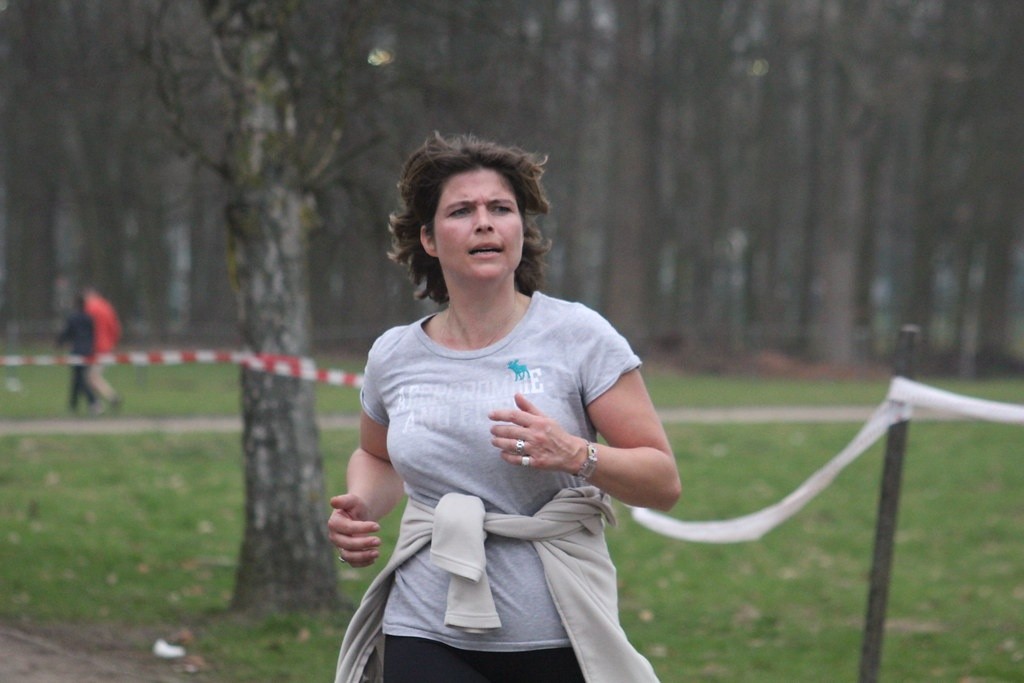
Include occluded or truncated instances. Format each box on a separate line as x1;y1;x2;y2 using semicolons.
574;439;597;481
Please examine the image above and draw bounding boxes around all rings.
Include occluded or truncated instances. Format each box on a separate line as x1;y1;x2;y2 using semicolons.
521;456;529;466
516;440;524;455
338;555;345;563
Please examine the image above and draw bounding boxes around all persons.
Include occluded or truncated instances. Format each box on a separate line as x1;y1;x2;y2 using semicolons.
326;130;681;683
53;287;120;414
